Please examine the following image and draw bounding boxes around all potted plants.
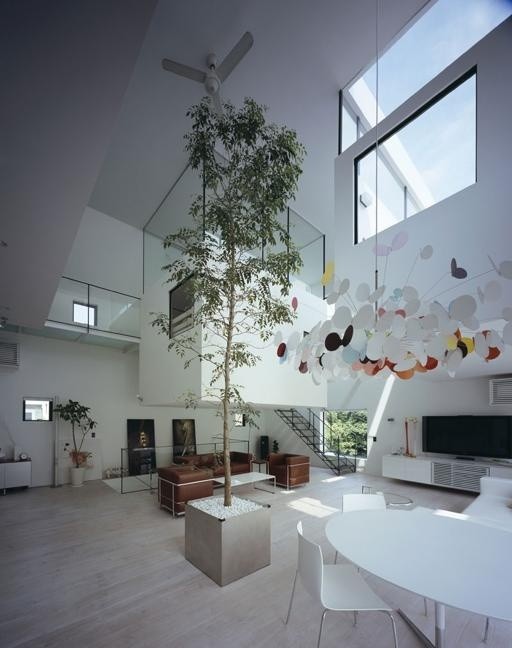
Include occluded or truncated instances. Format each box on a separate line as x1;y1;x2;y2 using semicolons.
148;95;307;587
53;399;98;487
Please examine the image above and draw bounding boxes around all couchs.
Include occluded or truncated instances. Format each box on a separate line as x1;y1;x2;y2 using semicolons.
158;465;213;519
269;454;309;491
175;451;253;479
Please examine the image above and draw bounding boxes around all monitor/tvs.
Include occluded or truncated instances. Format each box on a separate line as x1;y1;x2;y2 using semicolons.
422;415;512;461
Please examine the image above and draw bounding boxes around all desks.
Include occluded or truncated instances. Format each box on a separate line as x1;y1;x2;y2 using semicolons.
325;510;512;648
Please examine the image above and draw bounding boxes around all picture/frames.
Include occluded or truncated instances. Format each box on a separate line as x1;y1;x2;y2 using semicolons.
172;419;197;463
128;419;156;476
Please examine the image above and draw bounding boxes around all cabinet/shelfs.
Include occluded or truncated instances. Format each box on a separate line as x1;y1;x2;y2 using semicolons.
0;459;32;496
382;454;512;493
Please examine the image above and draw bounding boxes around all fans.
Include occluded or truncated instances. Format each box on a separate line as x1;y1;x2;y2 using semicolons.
161;31;254;115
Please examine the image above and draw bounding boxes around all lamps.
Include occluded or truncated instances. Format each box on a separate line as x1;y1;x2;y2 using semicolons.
274;1;512;386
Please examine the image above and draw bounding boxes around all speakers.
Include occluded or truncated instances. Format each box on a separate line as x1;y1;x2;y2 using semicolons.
260;436;269;461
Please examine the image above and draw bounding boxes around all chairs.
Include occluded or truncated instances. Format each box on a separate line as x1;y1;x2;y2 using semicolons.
285;520;398;648
334;494;387;573
